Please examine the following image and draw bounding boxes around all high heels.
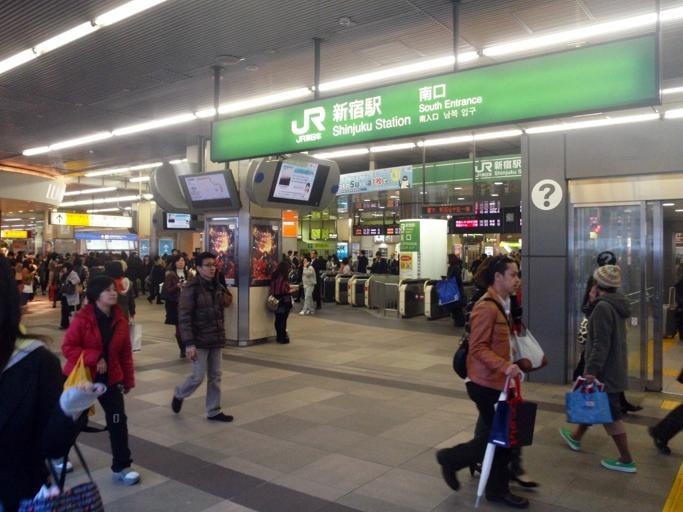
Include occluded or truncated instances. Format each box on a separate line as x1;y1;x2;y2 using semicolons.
509;468;541;488
468;460;484;476
621;400;644;416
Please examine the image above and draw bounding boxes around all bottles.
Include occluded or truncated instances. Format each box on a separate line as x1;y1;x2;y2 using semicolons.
179;275;185;292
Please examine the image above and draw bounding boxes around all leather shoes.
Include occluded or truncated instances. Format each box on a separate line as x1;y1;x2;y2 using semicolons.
647;425;671;456
435;446;460;491
483;491;530;508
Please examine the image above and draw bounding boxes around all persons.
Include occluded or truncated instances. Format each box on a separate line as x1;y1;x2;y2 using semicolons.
50;277;140;485
283;250;398;315
573;251;643;414
0;255;87;512
0;243;226;359
437;258;529;506
648;278;683;456
172;253;234;422
559;264;637;472
441;248;523;326
464;256;540;487
269;260;297;344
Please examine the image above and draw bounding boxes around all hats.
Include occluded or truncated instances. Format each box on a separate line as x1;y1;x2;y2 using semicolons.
591;264;624;288
85;275;116;303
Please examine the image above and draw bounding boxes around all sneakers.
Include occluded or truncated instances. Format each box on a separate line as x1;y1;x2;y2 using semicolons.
206;412;234;423
557;426;581;451
51;456;74;473
110;467;140;485
170;395;183;414
599;456;637;473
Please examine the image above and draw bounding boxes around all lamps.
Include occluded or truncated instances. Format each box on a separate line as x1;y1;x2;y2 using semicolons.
0;0;683;213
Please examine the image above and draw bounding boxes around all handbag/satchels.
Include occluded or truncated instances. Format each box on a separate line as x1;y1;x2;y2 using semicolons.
15;435;106;512
564;379;615;425
434;277;461;307
127;318;143;353
488;375;537;449
266;294;281;312
20;283;34;294
508;320;549;373
451;336;471;380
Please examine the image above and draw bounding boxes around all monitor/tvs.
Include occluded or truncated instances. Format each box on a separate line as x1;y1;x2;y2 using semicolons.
163;211;192;230
268;159;330;207
178;168;241;212
